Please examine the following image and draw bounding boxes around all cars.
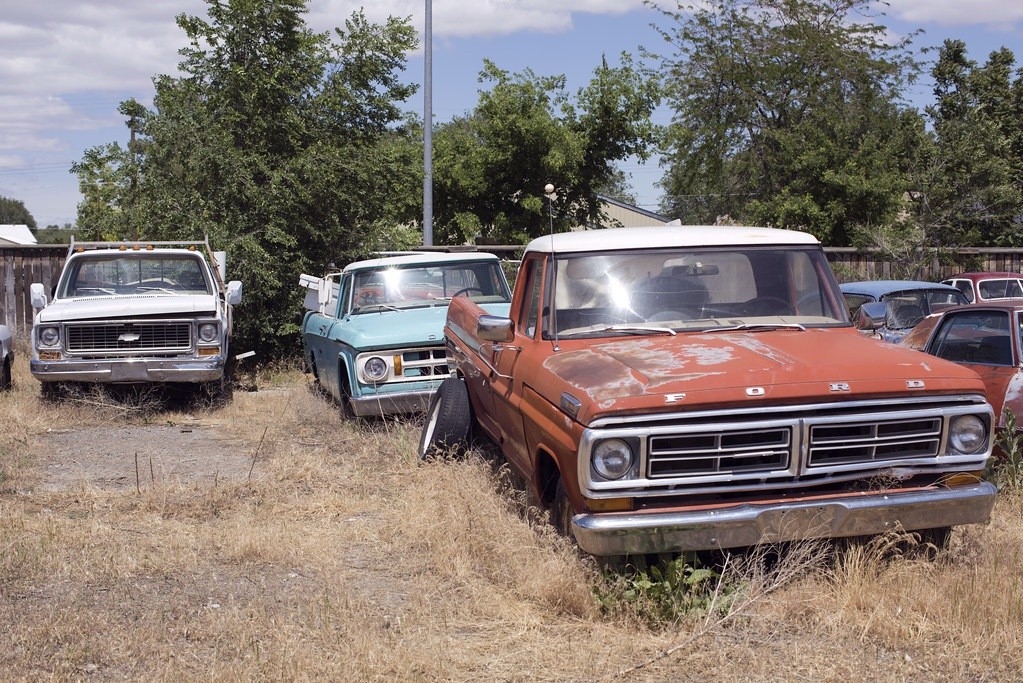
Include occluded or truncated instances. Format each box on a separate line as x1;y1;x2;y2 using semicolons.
893;299;1023;462
925;272;1023;315
446;223;996;572
0;325;16;391
787;279;986;363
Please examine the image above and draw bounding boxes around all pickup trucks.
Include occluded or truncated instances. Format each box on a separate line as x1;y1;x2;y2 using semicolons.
29;235;245;403
298;250;518;423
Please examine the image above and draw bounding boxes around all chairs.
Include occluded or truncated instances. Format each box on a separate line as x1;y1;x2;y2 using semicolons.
978;335;1012;365
896;305;926;327
636;276;711;323
